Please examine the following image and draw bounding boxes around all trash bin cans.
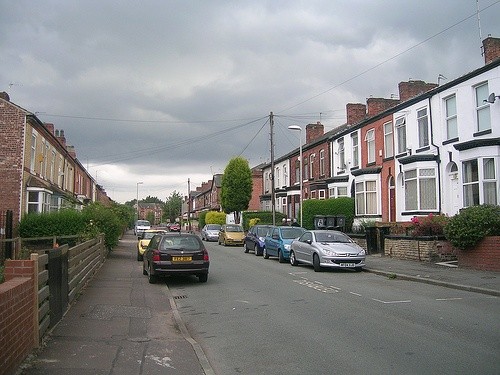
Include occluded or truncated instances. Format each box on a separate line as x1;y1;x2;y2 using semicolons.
336;214;346;232
312;214;326;230
325;215;336;230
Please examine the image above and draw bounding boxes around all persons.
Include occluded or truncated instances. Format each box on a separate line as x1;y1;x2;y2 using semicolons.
291;218;300;227
280;217;293;226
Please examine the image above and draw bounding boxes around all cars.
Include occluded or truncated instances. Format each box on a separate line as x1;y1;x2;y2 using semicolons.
137;229;168;261
263;226;307;264
166;222;181;232
244;225;273;256
143;232;210;281
201;224;223;241
218;224;246;247
291;230;366;272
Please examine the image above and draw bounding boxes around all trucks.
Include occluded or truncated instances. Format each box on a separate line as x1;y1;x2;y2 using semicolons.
134;220;152;235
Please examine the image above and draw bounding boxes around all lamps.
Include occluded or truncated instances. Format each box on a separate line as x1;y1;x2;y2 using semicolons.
483;93;500;103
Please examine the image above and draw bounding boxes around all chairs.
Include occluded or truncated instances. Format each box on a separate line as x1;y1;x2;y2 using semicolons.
180;239;188;245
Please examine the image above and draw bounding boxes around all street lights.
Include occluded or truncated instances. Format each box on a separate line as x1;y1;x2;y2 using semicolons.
289;125;303;228
137;181;143;220
